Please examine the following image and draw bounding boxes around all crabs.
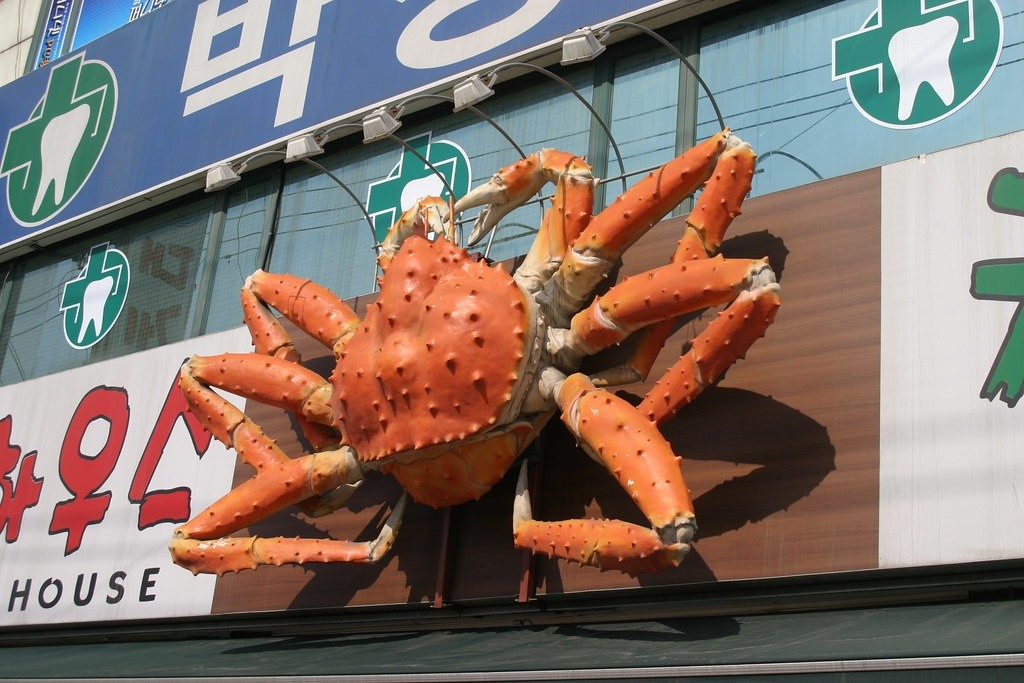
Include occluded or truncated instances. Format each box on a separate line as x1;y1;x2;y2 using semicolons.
168;127;781;580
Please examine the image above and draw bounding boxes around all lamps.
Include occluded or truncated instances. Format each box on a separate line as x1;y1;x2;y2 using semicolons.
453;62;626;193
561;21;725;132
285;123;463;248
363;92;543;230
205;147;382;293
